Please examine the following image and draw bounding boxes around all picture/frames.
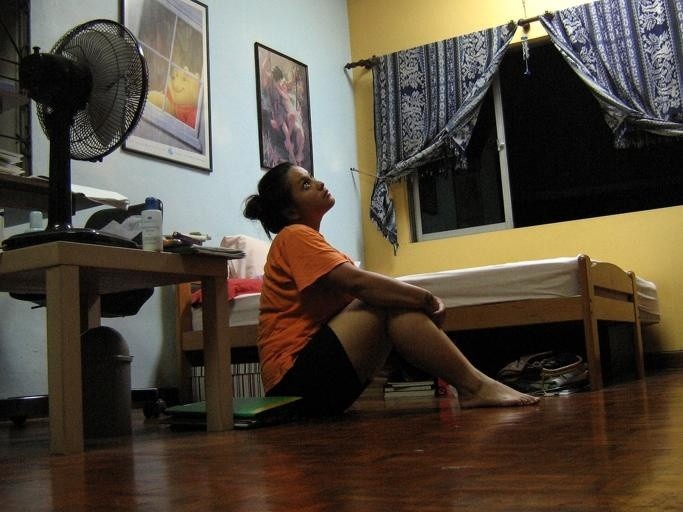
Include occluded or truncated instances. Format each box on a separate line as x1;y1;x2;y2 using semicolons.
256;42;315;177
117;1;213;172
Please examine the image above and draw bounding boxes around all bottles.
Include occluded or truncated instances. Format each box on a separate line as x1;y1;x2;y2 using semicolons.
141;197;163;252
26;211;43;233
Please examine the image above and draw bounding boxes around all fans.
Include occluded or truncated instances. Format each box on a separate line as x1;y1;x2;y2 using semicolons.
0;19;148;246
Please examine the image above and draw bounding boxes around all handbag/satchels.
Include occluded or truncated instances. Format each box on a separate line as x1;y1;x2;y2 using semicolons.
496;351;591;397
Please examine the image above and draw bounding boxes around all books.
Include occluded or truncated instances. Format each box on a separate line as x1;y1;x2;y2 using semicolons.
357;371;437;402
0;149;26;176
164;396;302;428
173;244;245;259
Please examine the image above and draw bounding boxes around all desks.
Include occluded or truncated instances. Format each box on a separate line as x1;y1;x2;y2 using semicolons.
1;239;233;458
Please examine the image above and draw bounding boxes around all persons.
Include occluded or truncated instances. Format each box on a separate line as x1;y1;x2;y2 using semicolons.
261;65;304;166
242;163;540;419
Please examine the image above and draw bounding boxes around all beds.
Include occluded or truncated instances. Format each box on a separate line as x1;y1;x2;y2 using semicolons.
177;252;661;391
